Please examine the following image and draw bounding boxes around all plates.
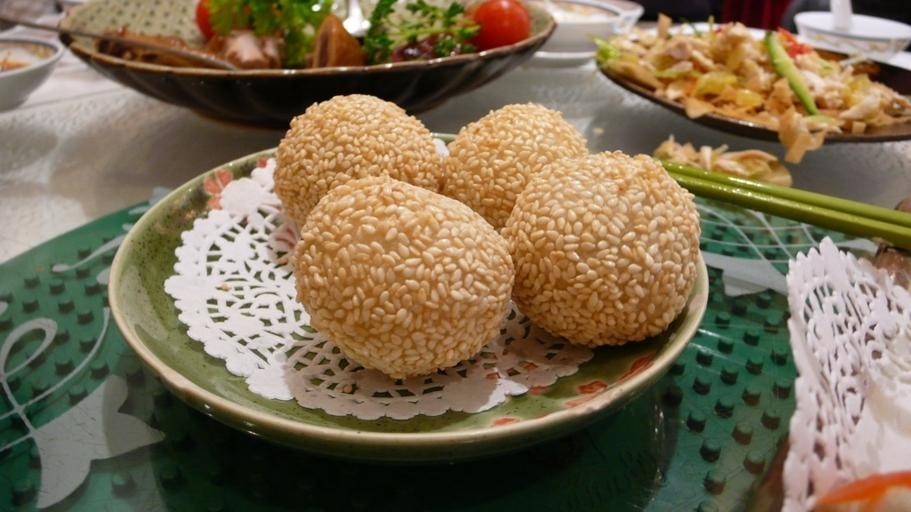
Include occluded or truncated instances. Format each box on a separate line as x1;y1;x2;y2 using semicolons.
107;130;709;466
55;0;558;126
595;20;911;144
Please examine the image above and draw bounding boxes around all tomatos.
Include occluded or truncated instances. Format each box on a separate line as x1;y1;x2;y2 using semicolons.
466;2;531;52
192;2;251;38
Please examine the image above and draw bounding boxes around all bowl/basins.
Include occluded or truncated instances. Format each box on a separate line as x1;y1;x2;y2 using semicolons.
0;38;63;103
533;0;643;70
795;10;911;64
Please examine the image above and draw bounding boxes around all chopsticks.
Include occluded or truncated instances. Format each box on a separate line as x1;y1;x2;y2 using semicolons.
658;160;911;252
